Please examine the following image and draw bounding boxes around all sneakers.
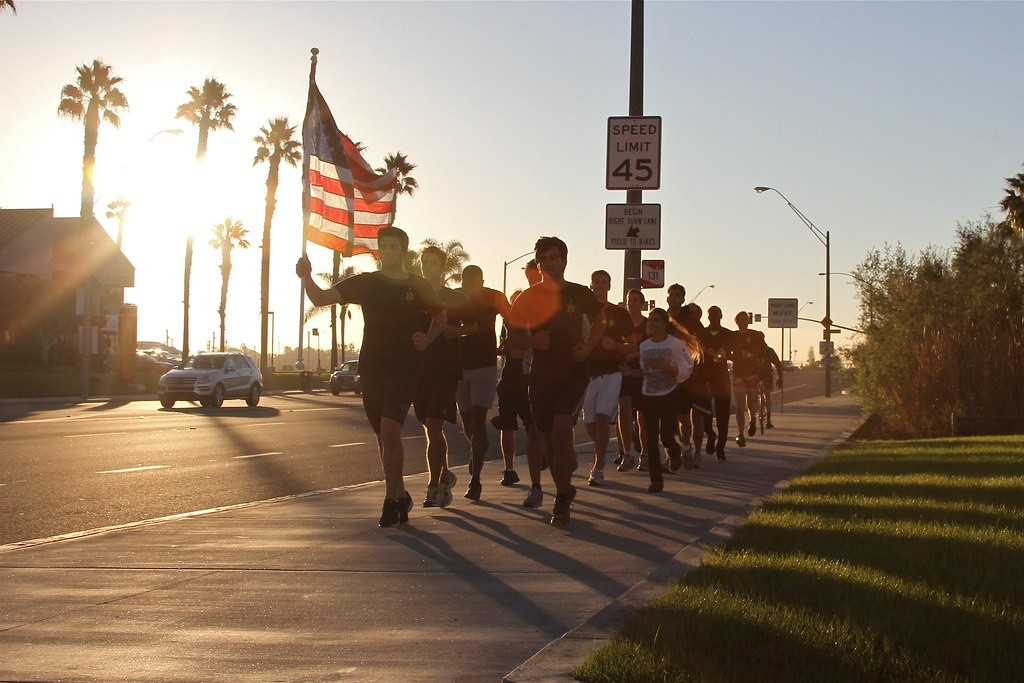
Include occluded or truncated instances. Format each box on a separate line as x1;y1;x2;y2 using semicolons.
468;448;472;476
570;449;578;472
583;415;776;493
423;482;438;506
523;486;542;508
568;486;577;501
500;470;520;485
438;470;457;507
379;491;413;526
464;481;482;501
551;508;570;529
541;452;549;473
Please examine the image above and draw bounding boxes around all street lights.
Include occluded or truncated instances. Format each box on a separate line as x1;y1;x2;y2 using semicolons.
818;272;873;330
754;186;831;398
790;302;814;362
268;311;275;367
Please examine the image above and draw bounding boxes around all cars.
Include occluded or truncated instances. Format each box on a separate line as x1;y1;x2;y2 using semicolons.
782;360;794;373
135;347;194;394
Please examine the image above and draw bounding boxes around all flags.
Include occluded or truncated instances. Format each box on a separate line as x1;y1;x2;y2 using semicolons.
298;79;399;261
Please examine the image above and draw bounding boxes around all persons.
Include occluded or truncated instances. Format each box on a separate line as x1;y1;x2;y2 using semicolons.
292;228;783;525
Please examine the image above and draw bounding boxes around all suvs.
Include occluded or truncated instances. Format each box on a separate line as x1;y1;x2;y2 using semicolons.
157;352;263;410
329;360;363;396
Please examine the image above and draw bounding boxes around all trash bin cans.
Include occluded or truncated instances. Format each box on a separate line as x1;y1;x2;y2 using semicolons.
300;371;313;391
